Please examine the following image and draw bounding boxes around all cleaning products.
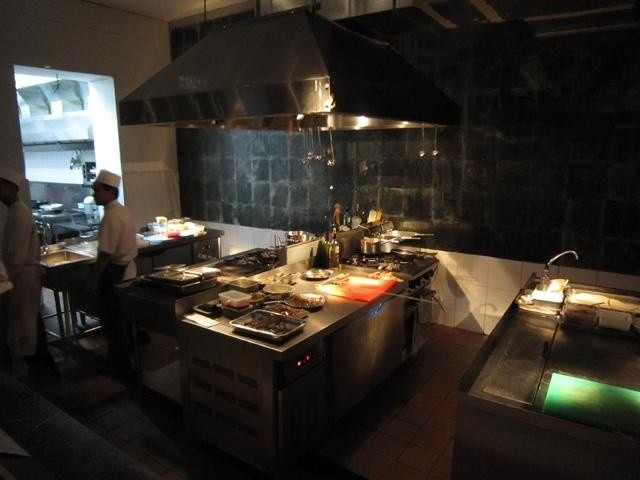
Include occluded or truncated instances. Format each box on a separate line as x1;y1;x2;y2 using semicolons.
332;203;342;226
323;233;340;266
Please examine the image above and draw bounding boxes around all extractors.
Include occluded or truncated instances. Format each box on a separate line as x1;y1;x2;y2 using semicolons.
120;0;457;131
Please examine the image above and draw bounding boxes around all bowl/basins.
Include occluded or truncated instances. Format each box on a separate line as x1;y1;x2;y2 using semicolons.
263;284;295;300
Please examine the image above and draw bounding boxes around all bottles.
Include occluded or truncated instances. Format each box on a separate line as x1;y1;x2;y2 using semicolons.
154;216;188;239
328;201;369;228
330;227;339;266
320;230;334;267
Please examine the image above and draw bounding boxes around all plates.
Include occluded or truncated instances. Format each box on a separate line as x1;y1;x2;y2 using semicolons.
302;270;330;280
283;293;327;309
40;202;64;212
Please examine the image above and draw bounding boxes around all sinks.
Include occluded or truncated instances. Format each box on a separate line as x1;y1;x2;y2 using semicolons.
567;284;639;314
40;248;96;292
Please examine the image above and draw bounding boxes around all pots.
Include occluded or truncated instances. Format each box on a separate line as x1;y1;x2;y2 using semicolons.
359;228;439;263
286;229;314;246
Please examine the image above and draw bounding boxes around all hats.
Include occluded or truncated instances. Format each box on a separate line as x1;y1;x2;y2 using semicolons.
97;170;121;189
0;165;21;186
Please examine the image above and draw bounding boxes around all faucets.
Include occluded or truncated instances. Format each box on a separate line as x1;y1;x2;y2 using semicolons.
546;249;579;268
43;222;52;246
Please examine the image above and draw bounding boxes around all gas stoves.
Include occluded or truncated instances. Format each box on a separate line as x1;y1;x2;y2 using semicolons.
217;248;276;276
352;252;438;290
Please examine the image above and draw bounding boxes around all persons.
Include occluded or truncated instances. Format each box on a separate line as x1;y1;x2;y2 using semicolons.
0;165;54;380
92;168;142;384
